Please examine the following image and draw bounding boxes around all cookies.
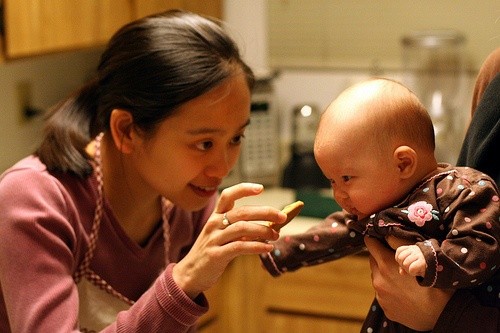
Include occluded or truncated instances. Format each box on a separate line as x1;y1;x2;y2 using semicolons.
257;201;304;242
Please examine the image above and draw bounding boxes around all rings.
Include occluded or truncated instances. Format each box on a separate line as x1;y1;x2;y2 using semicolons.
223;213;230;225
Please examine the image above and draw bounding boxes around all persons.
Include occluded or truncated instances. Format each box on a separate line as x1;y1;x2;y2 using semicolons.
259;79;500;333
365;73;500;333
0;9;287;333
